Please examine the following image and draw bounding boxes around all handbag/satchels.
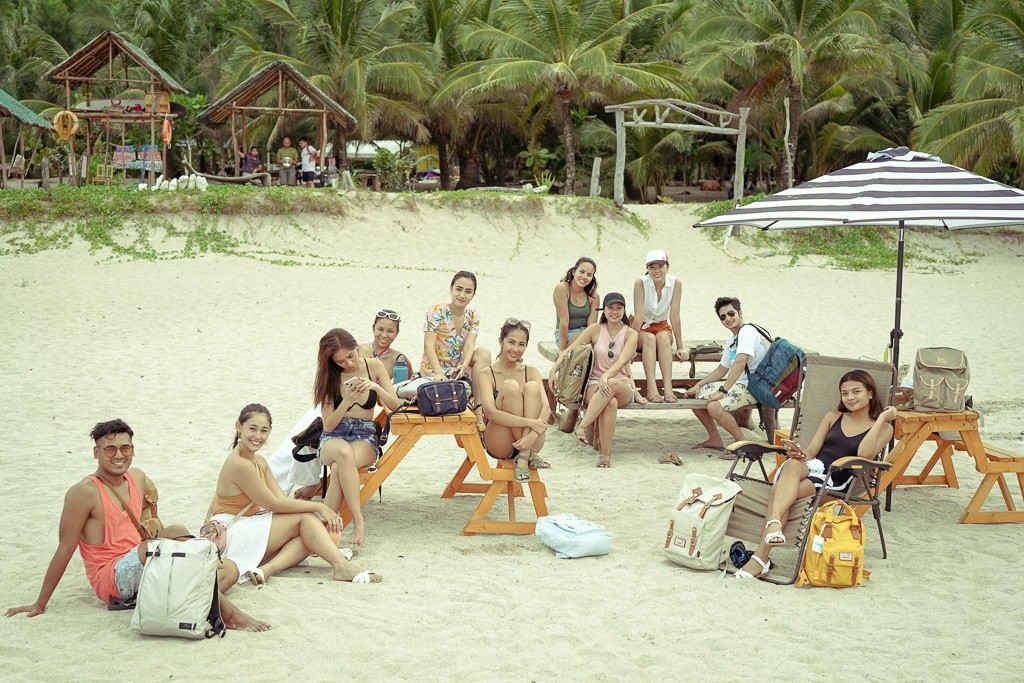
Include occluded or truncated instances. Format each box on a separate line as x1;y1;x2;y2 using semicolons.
291;417;323;462
200;520;230;557
131;534;226;640
287;445;321;486
417;380;468;417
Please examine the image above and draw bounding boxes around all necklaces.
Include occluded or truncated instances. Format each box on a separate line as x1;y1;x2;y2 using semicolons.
371;341;392;359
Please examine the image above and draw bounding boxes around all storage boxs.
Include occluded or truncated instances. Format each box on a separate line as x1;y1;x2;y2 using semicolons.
115;144;161;161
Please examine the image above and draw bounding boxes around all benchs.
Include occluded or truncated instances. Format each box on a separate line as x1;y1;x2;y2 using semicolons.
960;436;1024;524
540;377;803;407
317;430;551;535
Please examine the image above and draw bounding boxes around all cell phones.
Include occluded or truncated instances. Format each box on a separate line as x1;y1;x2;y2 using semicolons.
781;439;806;457
344;377;362;391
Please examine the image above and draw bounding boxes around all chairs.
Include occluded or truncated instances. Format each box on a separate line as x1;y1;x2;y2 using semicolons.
719;356;897;586
91;165;113;186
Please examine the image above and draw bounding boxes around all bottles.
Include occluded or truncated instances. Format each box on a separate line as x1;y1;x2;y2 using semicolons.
394;354;408;384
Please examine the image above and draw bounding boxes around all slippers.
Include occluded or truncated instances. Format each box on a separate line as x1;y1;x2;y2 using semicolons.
340;548;358;561
718;453;737;460
729;541;774;570
691;444;726;451
247;567;266;585
339;568;384;583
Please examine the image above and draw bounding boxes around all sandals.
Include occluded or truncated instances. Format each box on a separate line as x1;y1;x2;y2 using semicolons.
571;426;589;447
513;448;552;483
765;520;786;546
596;453;611;468
731;554;770;581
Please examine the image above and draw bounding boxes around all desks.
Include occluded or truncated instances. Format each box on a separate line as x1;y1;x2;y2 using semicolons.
890;404;988;488
537;340;726;446
333;403;499;538
111;160;163;186
357;173;383;192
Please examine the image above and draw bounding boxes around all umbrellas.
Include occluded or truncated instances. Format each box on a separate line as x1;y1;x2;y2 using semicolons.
691;144;1024;511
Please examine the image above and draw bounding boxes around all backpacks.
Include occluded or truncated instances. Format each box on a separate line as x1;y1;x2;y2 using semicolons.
913;346;971;412
535;512;610;559
664;473;744;570
794;500;872;589
556;344;595;405
736;323;807;410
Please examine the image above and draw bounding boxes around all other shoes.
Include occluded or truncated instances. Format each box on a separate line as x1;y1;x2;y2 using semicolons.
647;390;678;403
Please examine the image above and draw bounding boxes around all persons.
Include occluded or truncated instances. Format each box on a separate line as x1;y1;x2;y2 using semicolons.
357;310;412;406
733;369;897;579
239;147;263;186
478;319;551;481
552;256;599;354
686;298;772;451
548;293;647;468
728;169;754;199
276;136;317;189
399;165;424;193
420;270;491;433
209;404;384;584
58;155;66;185
3;419;273;632
625;169;648;199
631;249;689;402
315;327;400;546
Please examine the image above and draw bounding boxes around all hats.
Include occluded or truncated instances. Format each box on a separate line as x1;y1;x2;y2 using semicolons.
645;249;668;268
603;293;625;307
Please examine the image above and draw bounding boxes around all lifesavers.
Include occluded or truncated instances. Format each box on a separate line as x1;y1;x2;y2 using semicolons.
53;110;79;136
160;119;173;144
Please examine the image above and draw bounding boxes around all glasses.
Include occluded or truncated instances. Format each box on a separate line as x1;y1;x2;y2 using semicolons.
102;444;134;458
720;310;736;321
608;341;616;358
505;318;531;333
377;311;400;321
